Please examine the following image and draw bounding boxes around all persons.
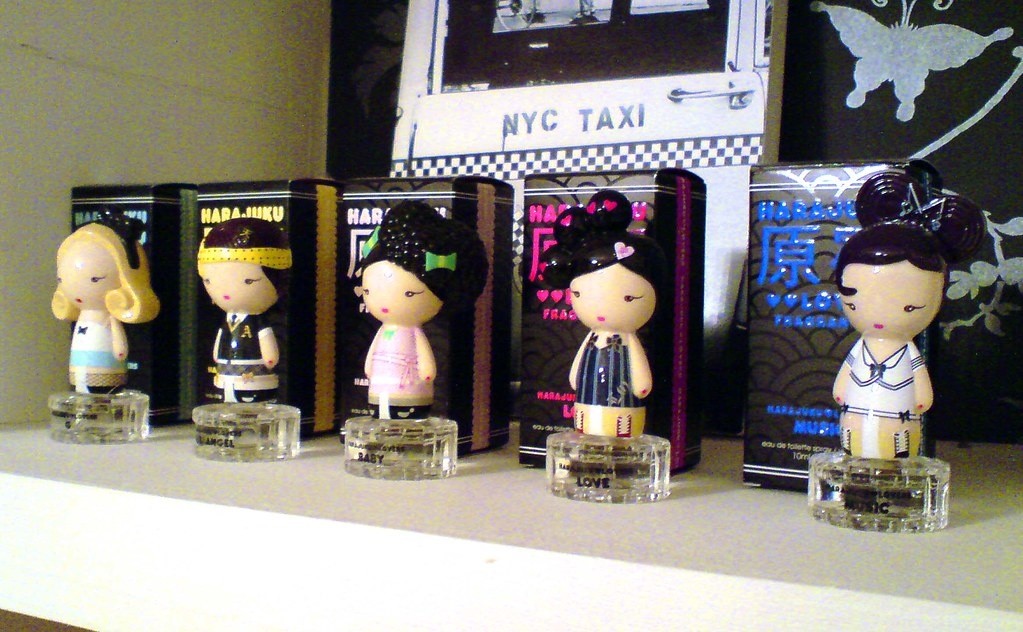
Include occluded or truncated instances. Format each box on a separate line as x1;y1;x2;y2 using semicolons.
360;200;488;419
832;158;988;460
50;208;161;392
197;217;292;403
539;190;670;437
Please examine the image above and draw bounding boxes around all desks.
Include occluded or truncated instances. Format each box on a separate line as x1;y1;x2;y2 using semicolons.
0;423;1023;632
